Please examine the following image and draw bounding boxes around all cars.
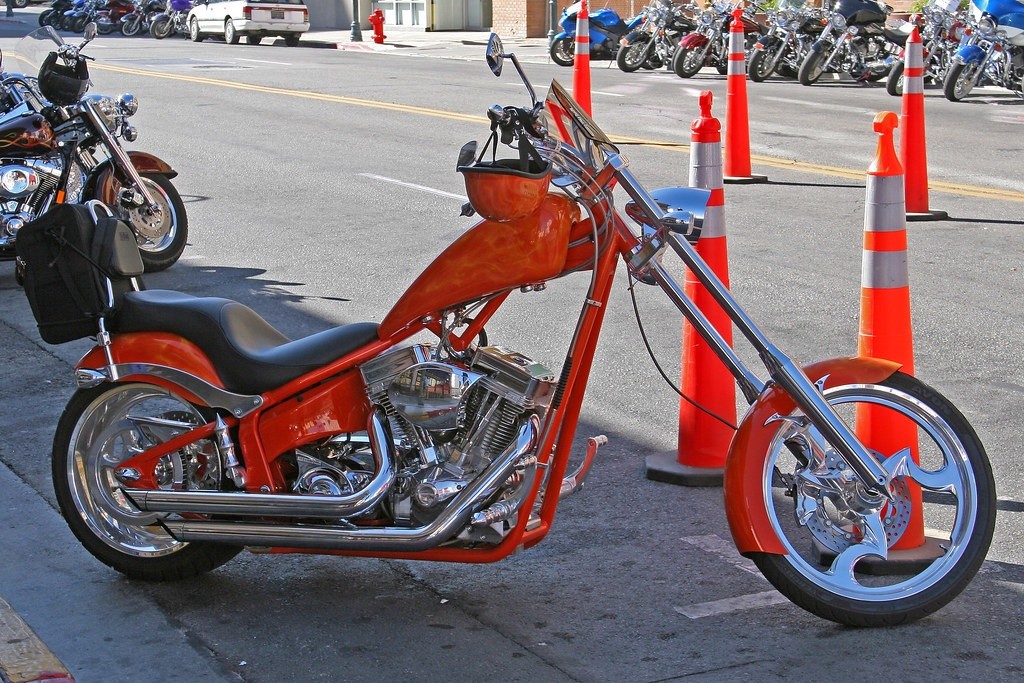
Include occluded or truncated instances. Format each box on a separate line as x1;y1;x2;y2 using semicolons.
185;0;311;47
4;0;47;9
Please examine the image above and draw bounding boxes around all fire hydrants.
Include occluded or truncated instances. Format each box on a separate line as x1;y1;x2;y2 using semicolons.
368;8;387;45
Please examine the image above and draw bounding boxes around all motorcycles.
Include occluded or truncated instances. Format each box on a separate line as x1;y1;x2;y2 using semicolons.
672;0;798;79
617;1;728;75
14;36;998;629
796;0;945;88
39;0;225;40
0;21;189;273
886;0;1024;103
550;1;663;71
747;1;893;84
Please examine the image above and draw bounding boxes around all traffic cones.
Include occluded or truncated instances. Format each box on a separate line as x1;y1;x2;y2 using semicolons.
722;8;769;185
808;110;950;574
645;90;739;487
899;27;950;221
572;0;593;119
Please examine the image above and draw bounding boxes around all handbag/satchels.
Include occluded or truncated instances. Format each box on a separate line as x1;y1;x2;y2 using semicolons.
15;204;147;345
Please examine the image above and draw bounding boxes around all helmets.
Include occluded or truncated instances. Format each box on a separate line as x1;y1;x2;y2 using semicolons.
38;51;89;105
458;158;553;223
